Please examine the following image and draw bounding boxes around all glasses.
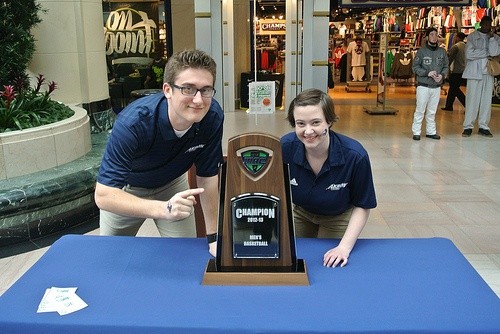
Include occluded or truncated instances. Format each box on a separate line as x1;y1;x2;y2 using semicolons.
168;83;216;98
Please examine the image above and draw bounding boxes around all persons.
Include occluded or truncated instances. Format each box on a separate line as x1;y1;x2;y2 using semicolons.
281;88;377;268
411;28;449;139
94;50;225;239
461;16;500;137
440;32;467;114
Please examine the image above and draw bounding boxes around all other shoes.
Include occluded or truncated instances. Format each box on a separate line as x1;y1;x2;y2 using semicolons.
462;129;472;137
441;107;453;111
478;128;493;137
426;135;440;139
413;135;420;140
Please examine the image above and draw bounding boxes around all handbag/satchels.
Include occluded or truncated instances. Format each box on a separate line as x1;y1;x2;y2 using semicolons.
487;56;500;76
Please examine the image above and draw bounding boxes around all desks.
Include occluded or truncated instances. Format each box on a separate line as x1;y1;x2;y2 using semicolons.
0;233;500;333
